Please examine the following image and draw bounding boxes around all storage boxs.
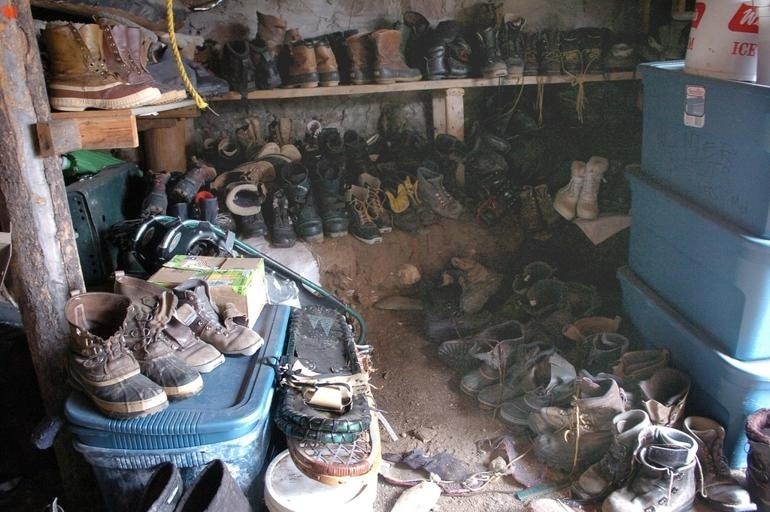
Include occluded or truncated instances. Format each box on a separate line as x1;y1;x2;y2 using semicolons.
624;164;770;362
637;59;768;240
623;268;770;472
60;294;296;511
146;252;269;330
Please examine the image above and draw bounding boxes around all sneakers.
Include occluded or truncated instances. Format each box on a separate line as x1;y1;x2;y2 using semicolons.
137;457;257;511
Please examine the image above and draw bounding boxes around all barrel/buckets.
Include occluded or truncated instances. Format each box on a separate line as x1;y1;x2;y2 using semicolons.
684;0;759;82
263;442;378;512
756;0;770;85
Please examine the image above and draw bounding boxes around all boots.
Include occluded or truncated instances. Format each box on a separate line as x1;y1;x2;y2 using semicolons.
143;116;641;247
48;2;690;112
419;246;769;510
64;268;265;419
